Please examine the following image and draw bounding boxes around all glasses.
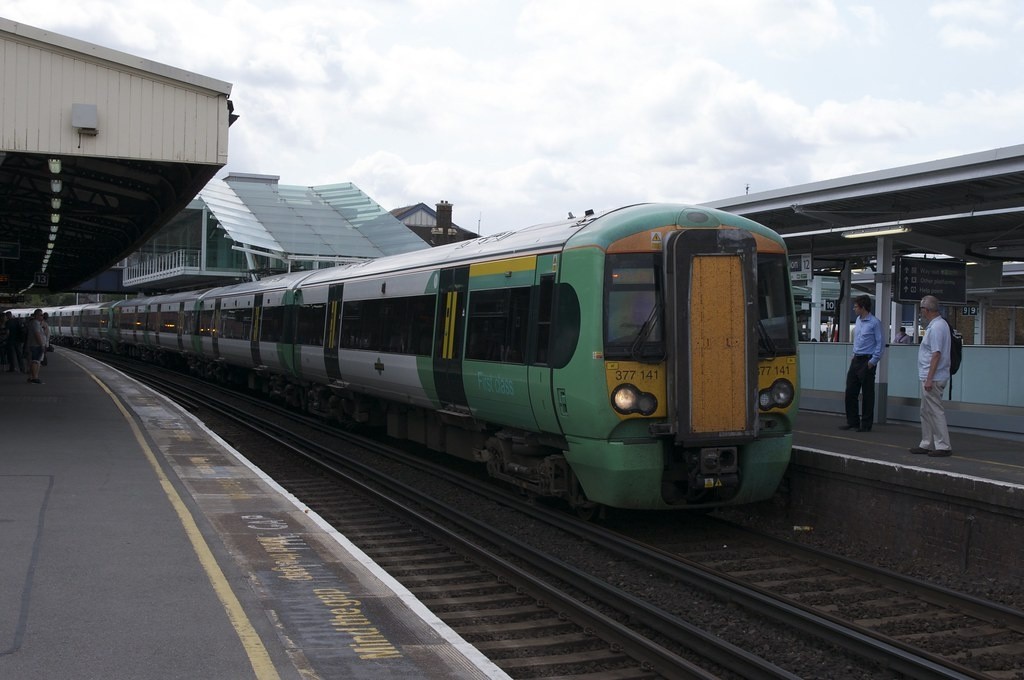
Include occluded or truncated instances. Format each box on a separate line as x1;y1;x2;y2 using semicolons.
919;306;926;309
853;306;859;308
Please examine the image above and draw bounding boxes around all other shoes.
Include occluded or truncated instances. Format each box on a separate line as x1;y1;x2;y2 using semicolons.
856;427;870;432
909;447;931;454
928;450;951;457
28;377;46;385
5;369;15;373
838;424;860;430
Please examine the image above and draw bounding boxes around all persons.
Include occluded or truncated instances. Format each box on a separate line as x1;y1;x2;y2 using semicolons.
911;295;954;458
842;295;884;430
0;309;51;384
798;325;912;346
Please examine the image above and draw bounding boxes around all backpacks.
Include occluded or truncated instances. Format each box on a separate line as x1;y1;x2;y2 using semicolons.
942;317;963;374
15;321;27;343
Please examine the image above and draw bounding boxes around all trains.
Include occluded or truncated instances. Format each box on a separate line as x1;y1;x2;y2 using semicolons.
4;202;803;511
